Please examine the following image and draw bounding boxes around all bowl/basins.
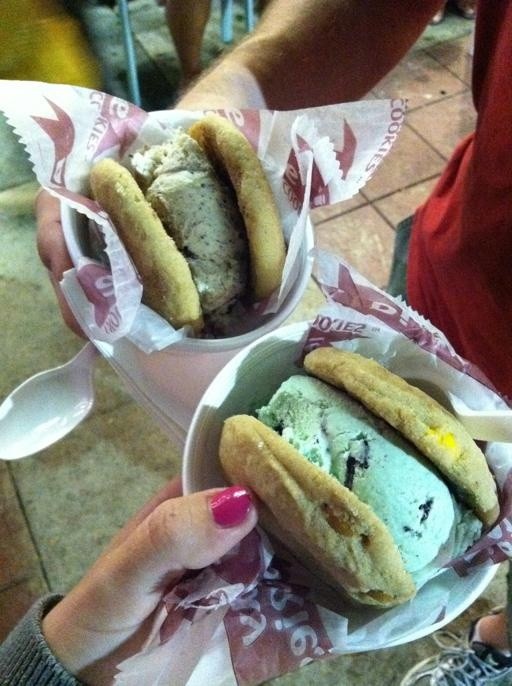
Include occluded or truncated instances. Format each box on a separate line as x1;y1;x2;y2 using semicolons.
179;316;511;657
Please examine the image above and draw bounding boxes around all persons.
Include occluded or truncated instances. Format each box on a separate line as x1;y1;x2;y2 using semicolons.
165;1;210;98
0;473;261;685
39;0;512;685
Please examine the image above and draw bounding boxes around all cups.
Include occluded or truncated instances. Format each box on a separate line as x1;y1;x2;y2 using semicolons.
57;112;313;355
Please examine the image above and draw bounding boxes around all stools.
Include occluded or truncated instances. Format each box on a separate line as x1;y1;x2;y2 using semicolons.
121;2;253;109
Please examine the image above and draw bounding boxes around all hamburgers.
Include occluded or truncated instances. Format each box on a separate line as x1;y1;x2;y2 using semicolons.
216;348;502;608
89;114;286;335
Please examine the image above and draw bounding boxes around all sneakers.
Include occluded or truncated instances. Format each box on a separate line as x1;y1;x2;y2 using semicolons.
399;602;512;685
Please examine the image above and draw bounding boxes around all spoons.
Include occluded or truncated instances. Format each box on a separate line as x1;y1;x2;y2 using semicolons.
0;340;101;461
